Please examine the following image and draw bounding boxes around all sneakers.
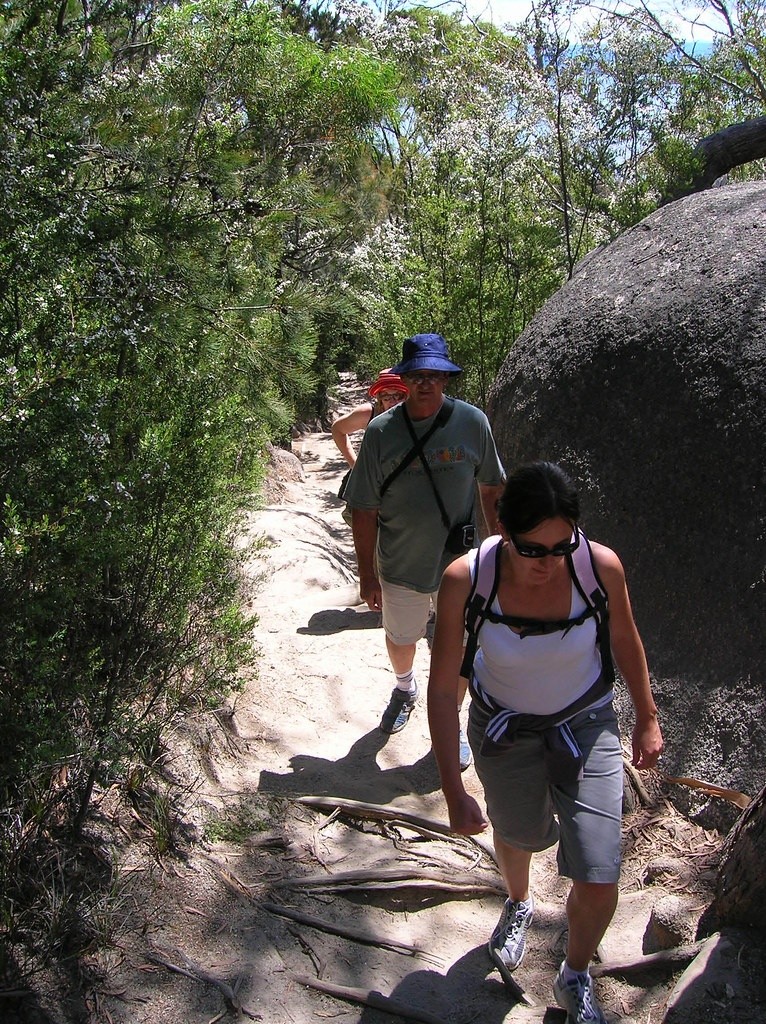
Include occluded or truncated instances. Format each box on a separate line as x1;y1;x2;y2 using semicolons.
459;723;476;768
489;894;534;970
553;956;607;1024
379;678;418;734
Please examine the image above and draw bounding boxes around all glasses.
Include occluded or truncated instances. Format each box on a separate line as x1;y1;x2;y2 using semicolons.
376;392;403;401
506;523;580;558
404;375;445;385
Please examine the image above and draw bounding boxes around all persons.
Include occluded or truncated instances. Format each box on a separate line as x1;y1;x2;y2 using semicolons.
424;456;667;1024
345;332;509;767
331;366;411;606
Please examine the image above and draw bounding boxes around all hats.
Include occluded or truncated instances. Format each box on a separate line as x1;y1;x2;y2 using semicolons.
368;368;411;398
388;333;462;377
